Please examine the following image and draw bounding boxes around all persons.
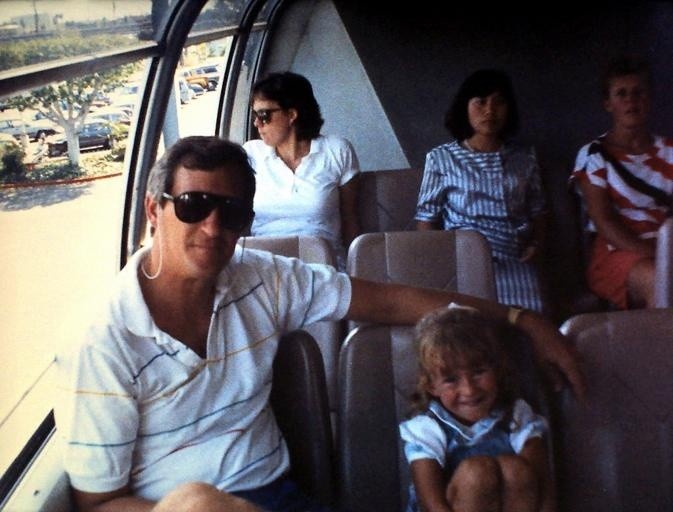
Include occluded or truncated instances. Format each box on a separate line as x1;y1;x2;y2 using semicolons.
567;53;673;310
393;302;555;511
48;133;597;511
413;62;557;332
235;71;364;279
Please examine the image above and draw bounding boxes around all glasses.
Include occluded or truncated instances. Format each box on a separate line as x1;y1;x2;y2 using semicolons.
252;107;283;125
163;191;253;231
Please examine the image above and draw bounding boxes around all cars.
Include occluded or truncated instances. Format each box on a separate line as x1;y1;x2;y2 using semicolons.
1;63;217;161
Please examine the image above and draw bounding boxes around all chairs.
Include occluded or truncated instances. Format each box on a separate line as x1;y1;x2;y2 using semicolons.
238;167;673;512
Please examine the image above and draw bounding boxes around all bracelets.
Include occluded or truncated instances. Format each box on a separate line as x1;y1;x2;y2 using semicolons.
505;304;527;324
